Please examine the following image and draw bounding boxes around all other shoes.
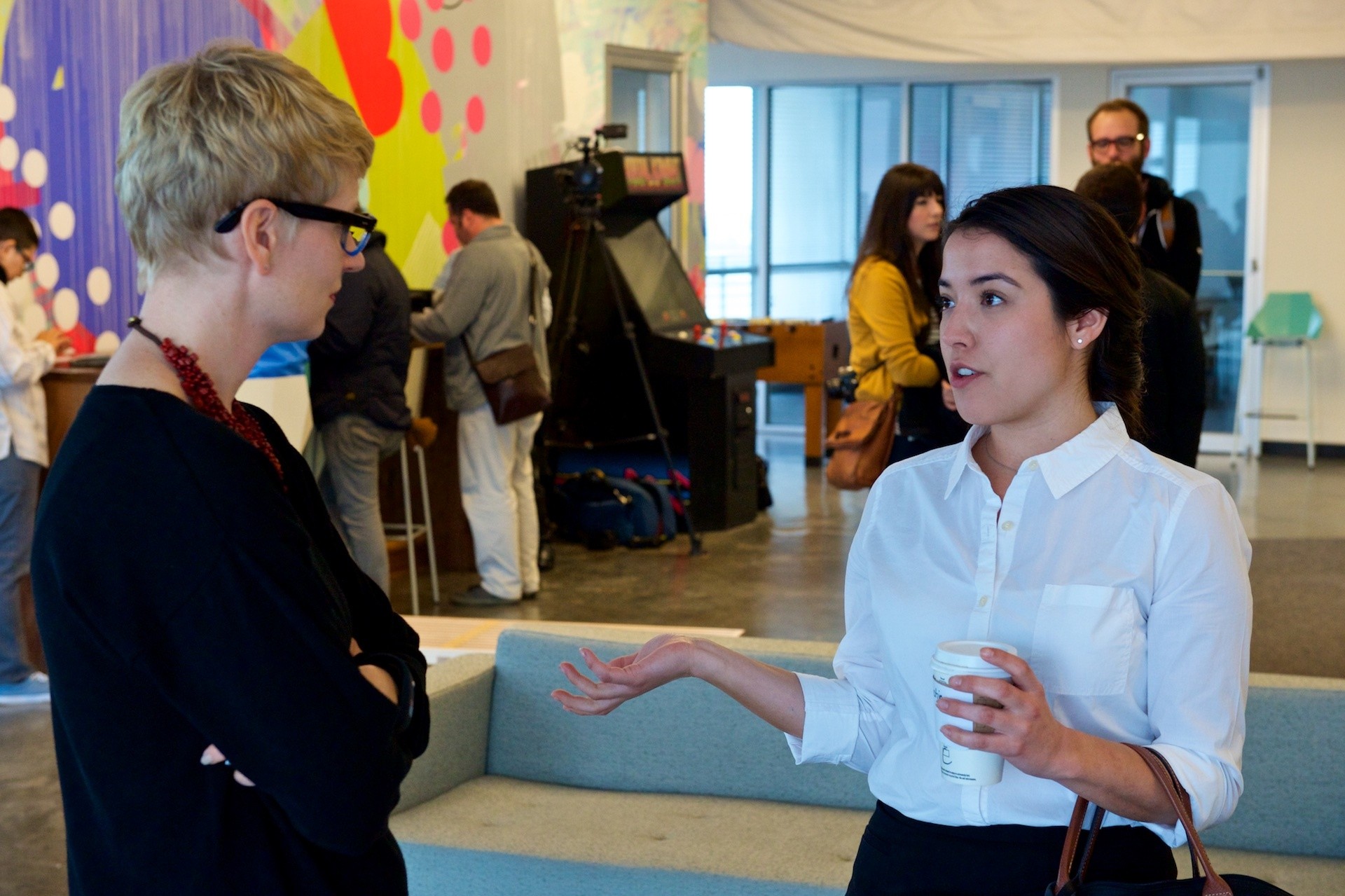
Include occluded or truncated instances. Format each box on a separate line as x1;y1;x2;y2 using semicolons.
467;585;537;600
450;587;520;606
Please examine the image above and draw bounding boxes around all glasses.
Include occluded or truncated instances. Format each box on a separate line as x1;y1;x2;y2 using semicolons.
215;198;377;255
1091;136;1145;151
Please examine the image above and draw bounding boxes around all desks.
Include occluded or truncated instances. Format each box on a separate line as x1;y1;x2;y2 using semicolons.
745;318;851;468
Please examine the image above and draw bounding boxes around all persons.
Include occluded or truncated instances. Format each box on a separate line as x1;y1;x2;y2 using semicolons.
0;202;55;702
26;38;434;894
405;177;551;607
1068;163;1207;470
552;183;1255;896
304;229;422;605
839;160;950;517
1084;96;1205;297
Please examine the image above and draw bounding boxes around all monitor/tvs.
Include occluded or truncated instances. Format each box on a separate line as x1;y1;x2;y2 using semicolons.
600;215;712;334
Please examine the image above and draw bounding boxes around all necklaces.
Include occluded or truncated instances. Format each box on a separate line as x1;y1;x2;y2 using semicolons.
127;313;283;483
982;434;1023;475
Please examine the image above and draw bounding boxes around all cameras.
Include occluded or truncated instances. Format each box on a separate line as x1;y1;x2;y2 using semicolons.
825;365;857;402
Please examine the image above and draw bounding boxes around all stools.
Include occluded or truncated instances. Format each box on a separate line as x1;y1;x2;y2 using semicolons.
374;435;440;617
1227;291;1323;471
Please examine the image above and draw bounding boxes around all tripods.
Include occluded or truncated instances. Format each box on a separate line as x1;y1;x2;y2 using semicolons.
538;123;705;555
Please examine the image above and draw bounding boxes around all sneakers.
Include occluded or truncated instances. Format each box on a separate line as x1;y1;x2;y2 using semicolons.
1;671;51;706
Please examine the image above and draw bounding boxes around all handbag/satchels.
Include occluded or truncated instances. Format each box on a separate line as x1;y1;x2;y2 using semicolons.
472;344;551;426
826;400;896;489
1049;742;1292;895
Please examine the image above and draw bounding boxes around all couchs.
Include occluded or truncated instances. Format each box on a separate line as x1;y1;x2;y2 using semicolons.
386;629;1344;895
